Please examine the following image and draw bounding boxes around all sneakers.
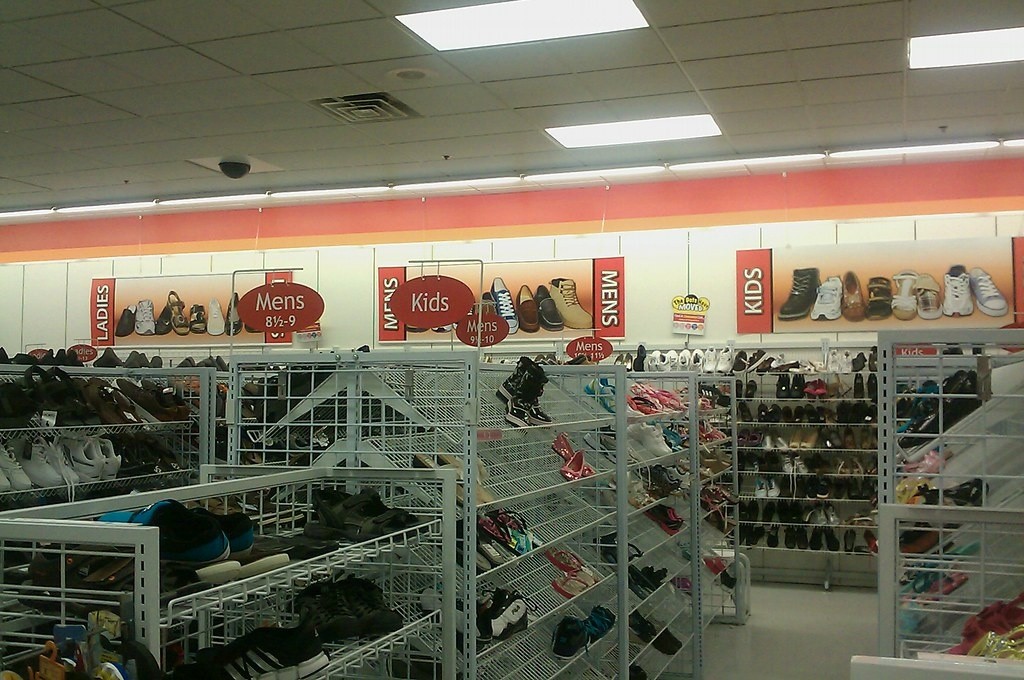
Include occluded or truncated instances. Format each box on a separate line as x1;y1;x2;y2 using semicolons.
0;347;1024;680
778;266;1008;321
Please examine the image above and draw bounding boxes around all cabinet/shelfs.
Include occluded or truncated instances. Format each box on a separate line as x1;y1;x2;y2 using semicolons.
0;328;1024;680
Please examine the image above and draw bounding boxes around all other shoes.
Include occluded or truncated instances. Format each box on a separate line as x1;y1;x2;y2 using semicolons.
406;277;595;335
115;291;263;337
